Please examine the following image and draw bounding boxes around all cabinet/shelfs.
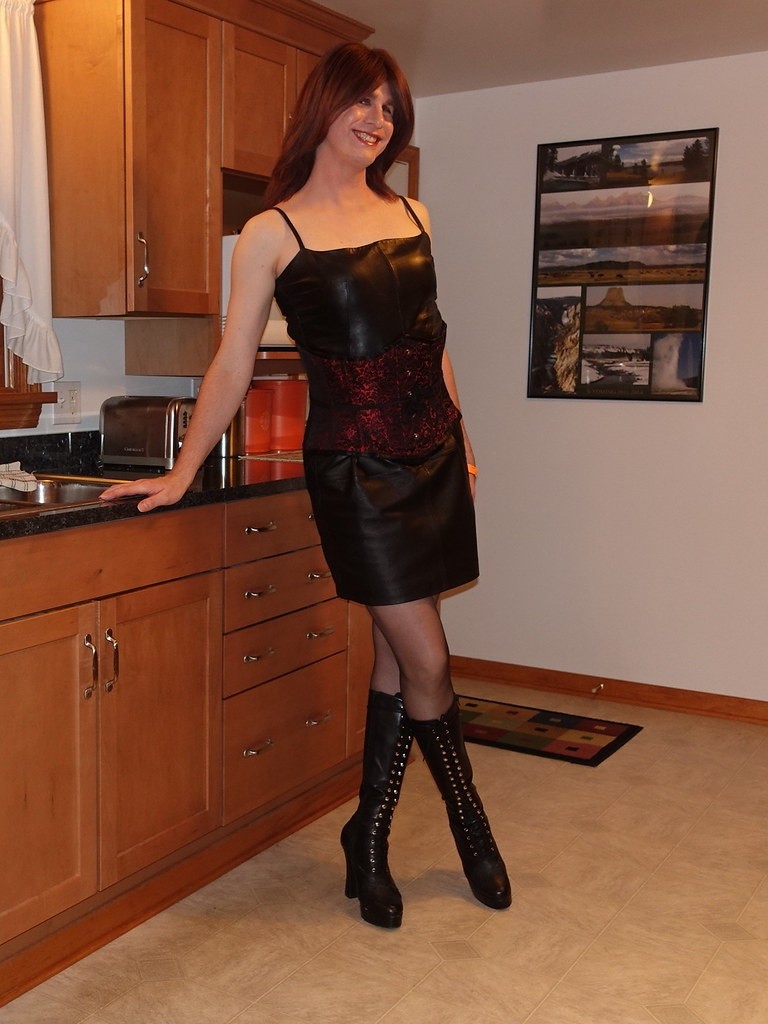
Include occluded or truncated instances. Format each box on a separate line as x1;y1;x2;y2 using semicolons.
0;442;378;1010
32;0;420;377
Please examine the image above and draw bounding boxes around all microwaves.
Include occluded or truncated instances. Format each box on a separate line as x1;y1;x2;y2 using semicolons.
221;235;297;347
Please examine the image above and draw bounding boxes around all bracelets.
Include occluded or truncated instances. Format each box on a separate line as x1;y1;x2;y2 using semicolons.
468;464;479;478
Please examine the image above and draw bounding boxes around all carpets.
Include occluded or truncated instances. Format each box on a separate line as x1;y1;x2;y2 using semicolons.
455;693;645;769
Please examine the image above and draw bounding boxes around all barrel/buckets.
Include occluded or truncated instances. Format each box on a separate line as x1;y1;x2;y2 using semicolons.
254;380;307;450
244;389;273;453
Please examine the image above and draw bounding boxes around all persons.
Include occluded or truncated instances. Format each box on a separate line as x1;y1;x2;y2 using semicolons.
98;42;515;930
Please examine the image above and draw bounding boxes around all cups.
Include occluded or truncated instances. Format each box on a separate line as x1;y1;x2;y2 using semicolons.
211;401;245;457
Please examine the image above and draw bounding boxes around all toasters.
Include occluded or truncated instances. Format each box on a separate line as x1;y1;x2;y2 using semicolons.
100;396;205;470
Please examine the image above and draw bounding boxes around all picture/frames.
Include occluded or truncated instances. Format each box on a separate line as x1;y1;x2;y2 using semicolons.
528;127;720;403
52;379;82;425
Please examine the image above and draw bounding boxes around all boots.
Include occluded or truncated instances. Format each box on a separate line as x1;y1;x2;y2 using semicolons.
405;692;512;911
340;690;417;927
